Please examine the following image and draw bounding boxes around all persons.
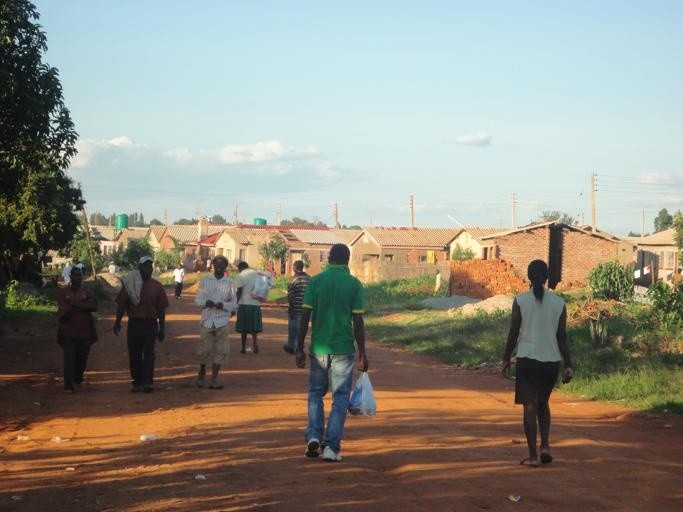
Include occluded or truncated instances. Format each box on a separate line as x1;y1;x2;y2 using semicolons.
234;262;264;354
75;262;85;286
500;259;574;467
192;256;203;273
295;243;368;463
194;255;238;391
672;268;682;293
432;269;442;297
171;262;184;298
107;261;115;274
281;260;314;355
114;264;121;273
112;254;169;396
58;259;73;289
56;268;99;395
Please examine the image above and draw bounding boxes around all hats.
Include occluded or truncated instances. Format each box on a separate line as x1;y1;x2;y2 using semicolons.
139;256;153;265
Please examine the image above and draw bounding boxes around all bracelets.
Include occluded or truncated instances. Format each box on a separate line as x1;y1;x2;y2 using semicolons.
358;354;365;358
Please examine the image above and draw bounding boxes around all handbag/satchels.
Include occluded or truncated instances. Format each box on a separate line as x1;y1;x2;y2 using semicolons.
252;271;272;302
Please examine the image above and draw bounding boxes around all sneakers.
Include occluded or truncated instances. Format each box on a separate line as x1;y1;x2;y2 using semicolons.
305;439;321;457
322;446;342;461
